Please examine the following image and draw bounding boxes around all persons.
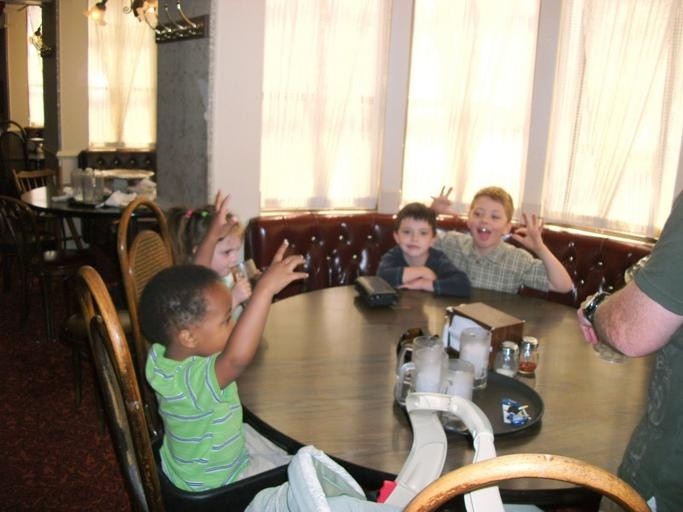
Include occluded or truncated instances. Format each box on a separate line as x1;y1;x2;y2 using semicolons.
138;190;308;497
577;191;683;511
377;202;470;297
169;204;252;323
427;187;573;295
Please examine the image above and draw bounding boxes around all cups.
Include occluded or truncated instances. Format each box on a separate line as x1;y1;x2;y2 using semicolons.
397;335;443;386
231;258;260;308
95;171;105;200
81;171;94;203
440;358;475;432
395;348;450;408
70;168;84;198
458;326;490;391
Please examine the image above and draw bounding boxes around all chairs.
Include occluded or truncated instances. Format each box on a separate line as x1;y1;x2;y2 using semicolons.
1;196;88;341
118;194;180;436
11;168;65;256
0;120;32;173
76;264;294;512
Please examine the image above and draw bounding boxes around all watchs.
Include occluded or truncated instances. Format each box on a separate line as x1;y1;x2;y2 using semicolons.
582;291;610;332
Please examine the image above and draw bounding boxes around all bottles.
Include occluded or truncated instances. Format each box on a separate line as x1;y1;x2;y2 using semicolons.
517;338;539;375
493;341;519;377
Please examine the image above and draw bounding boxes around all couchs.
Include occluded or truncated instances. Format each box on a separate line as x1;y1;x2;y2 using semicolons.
243;214;657;308
77;149;158;172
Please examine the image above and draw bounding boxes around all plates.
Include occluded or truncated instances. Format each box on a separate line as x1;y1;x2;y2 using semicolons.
403;369;548;438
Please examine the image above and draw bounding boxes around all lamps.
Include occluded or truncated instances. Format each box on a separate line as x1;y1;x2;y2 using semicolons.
82;0;108;26
29;22;55;58
125;1;156;22
